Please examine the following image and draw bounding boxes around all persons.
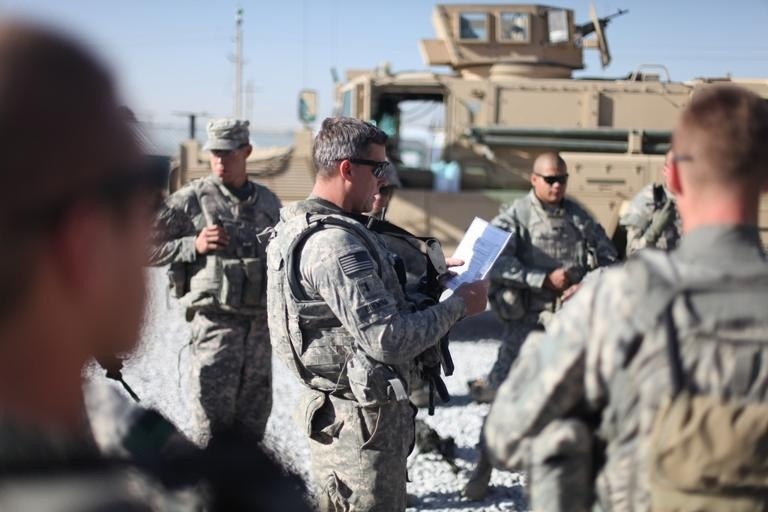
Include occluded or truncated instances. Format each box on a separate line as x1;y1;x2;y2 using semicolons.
1;28;224;511
143;118;284;448
258;118;487;512
461;152;620;502
618;147;684;257
366;158;444;411
482;84;767;512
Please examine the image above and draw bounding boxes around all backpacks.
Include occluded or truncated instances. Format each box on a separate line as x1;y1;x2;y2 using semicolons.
646;389;768;510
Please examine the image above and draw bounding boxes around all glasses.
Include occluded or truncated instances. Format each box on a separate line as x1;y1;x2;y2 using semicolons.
533;173;567;183
331;156;388;178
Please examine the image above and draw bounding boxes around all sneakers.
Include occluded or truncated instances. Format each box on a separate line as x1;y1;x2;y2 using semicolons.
464;453;492;500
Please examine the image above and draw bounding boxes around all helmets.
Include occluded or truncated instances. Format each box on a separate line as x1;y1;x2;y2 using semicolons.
380;158;402;190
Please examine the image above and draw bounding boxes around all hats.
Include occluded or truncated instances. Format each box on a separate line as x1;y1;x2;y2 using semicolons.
203;118;249;151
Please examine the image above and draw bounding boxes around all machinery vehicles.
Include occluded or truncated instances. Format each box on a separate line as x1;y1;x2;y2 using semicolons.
164;2;768;260
